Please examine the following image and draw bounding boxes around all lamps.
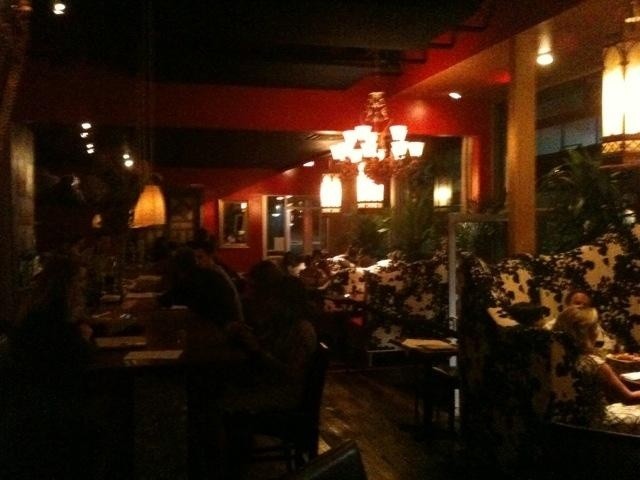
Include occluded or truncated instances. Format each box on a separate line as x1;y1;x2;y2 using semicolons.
597;33;640;171
327;87;428;162
355;164;386;211
317;170;345;215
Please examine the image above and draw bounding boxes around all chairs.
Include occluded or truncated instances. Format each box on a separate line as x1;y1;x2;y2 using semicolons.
300;438;367;479
415;362;458;433
214;340;328;480
348;317;403;367
544;410;639;479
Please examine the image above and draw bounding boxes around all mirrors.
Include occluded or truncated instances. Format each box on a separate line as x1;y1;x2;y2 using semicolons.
216;197;251;250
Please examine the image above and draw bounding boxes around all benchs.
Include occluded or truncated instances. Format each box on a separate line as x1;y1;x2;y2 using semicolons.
0;218;640;479
